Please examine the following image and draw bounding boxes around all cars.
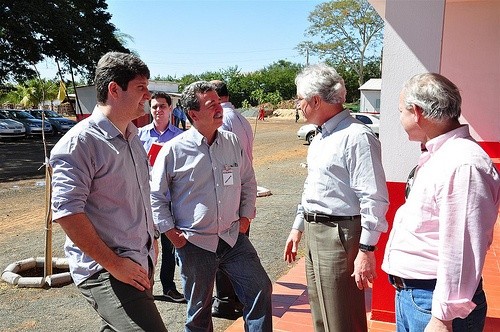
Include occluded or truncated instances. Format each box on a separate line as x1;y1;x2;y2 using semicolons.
296;113;380;145
5;109;54;141
0;109;26;142
21;109;78;139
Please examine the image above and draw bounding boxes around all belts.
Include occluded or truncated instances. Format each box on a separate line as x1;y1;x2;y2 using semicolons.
302;211;362;224
388;274;482;292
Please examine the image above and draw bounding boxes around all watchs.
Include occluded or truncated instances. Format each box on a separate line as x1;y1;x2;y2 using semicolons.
154;230;160;239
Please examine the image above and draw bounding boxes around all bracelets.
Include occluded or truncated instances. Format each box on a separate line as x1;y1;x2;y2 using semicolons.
359;244;379;252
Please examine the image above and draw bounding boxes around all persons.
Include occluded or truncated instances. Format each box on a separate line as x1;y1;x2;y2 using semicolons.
284;64;390;332
150;81;272;332
173;103;187;131
208;80;254;321
381;73;500;332
138;91;186;302
49;52;167;332
258;105;265;120
295;109;299;122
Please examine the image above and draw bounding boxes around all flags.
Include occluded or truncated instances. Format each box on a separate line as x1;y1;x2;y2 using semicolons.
57;81;67;102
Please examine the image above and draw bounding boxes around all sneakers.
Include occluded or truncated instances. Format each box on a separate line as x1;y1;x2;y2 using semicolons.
163;284;184;302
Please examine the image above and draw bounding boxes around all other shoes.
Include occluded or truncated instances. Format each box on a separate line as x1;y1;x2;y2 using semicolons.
211;305;244;320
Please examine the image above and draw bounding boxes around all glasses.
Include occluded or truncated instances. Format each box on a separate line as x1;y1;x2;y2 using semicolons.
405;165;420;199
